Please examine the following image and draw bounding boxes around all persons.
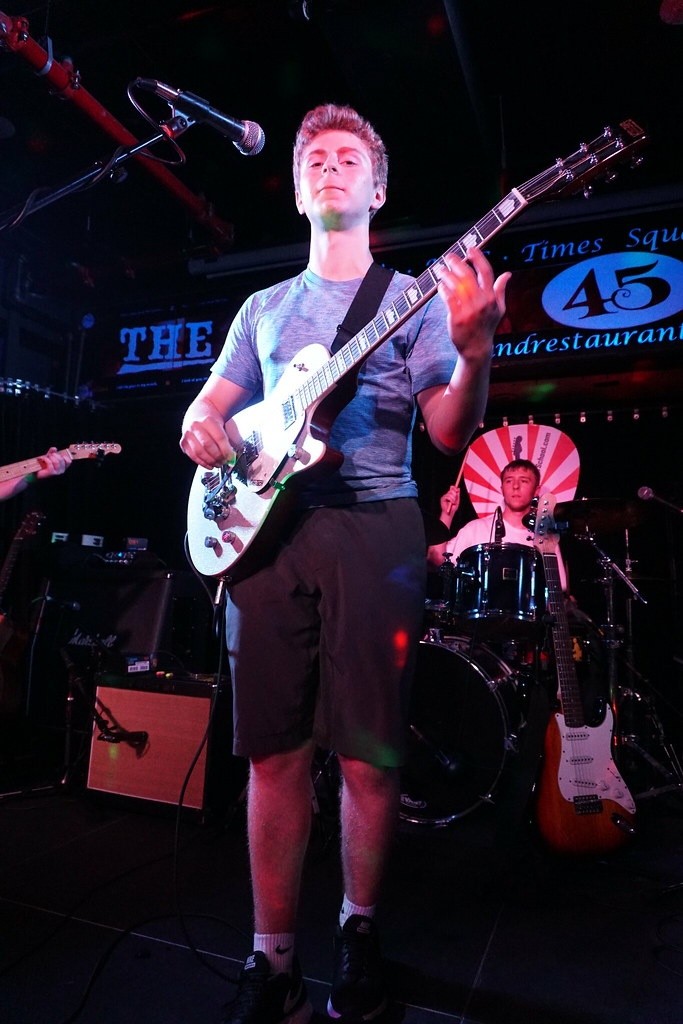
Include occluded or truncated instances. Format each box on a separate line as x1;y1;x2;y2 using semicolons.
428;459;568;592
0;447;72;501
179;104;512;1024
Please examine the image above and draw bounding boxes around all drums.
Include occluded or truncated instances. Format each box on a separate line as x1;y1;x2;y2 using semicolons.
334;632;522;834
452;540;544;631
421;564;468;628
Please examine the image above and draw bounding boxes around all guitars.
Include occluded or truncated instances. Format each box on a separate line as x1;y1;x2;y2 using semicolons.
0;438;122;485
0;510;49;651
526;493;640;852
187;117;654;587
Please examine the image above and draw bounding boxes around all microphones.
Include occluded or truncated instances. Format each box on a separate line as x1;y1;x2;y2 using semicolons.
638;486;683;513
103;731;147;743
496;507;506;539
137;77;265;156
443;757;465;780
46;596;81;611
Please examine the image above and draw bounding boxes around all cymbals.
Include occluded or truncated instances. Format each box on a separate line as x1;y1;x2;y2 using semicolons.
521;495;643;538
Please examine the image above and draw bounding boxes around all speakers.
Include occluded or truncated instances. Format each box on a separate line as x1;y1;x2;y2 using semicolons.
67;567;212;674
81;671;250;823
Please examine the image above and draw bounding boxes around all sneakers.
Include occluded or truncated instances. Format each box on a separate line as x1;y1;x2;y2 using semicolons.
215;950;314;1024
326;912;388;1023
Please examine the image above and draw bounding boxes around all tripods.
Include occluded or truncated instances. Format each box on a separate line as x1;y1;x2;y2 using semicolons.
613;586;683;807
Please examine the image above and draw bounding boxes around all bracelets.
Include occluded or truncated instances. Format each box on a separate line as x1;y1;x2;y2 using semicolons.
23;472;36;485
